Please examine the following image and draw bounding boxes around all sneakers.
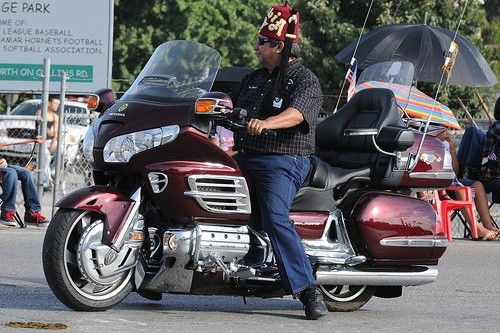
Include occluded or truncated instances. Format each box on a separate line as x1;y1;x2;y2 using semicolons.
24;211;50;227
0;210;16;226
300;286;329;320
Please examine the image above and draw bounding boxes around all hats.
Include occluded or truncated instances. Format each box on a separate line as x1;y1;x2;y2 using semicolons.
258;0;299;43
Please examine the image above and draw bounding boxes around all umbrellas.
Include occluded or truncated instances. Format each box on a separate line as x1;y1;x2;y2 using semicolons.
334;11;499;88
353;81;461;129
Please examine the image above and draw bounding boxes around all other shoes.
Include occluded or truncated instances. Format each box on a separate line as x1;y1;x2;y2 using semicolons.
43;185;53;192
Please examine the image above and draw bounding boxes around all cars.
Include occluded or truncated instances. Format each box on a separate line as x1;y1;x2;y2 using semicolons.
0;95;104;167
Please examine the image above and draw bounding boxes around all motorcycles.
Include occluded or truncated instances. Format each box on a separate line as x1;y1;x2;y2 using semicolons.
42;0;467;313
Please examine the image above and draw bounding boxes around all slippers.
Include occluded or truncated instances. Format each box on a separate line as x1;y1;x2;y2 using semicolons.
478;228;500;241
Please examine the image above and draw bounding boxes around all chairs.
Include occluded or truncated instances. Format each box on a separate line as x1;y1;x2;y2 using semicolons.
438;186;479;241
455;125;500;237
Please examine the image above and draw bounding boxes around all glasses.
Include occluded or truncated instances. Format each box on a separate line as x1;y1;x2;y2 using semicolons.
257;37;274;46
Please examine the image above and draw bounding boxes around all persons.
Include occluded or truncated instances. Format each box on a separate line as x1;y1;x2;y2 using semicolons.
35;96;60;186
0;157;49;227
427;121;500;241
204;5;330;320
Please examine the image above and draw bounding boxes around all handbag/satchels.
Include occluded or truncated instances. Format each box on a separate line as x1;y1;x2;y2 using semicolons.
481;152;496;168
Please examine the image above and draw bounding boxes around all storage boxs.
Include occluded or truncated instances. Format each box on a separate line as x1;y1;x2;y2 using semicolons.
399;133;455;188
339;188;448;266
